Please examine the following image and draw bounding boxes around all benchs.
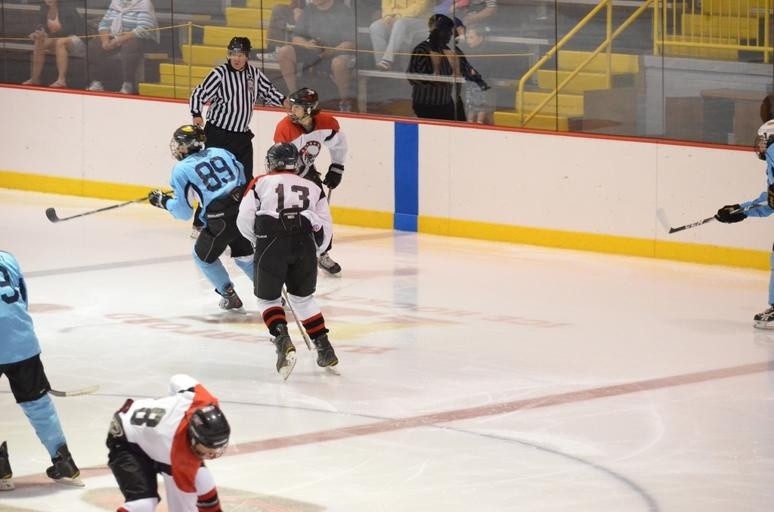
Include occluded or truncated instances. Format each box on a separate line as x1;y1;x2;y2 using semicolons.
245;1;688;125
0;0;245;120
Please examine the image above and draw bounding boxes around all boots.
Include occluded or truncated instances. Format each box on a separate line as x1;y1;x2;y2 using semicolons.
46;444;80;479
319;253;341;273
312;334;338;367
215;283;242;310
269;323;296;373
0;441;12;480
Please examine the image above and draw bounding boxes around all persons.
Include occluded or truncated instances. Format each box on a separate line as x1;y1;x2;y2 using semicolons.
21;0;87;88
86;0;160;95
189;37;292;231
147;125;286;314
0;250;86;489
106;373;231;512
714;119;773;332
256;0;515;125
273;88;348;274
235;140;341;380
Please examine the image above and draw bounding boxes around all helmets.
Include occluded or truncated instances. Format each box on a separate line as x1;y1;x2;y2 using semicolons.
264;142;298;174
226;36;251;61
170;125;206;161
287;87;321;126
187;405;230;460
754;120;774;161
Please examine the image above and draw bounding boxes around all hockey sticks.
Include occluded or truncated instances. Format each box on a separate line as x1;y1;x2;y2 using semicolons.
656;200;769;234
50;386;98;397
46;190;174;223
284;286;311;364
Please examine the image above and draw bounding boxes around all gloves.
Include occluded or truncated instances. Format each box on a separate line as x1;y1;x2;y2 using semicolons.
148;189;173;210
323;163;344;190
714;204;748;223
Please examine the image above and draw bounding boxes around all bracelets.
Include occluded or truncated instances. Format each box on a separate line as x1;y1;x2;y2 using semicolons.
113;35;122;48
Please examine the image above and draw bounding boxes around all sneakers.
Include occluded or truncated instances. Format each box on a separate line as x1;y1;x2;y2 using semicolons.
20;79;132;95
256;52;277;62
376;60;391;71
754;303;774;321
338;101;351;113
